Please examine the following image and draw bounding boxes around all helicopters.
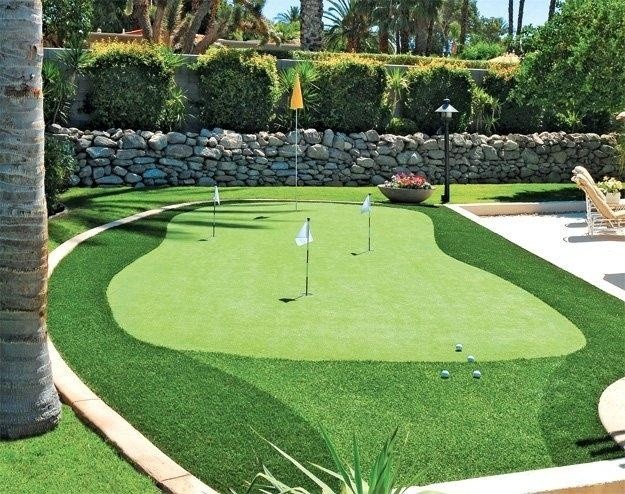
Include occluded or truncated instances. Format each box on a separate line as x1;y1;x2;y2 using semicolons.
473;371;480;377
467;356;475;363
456;344;462;350
441;370;449;378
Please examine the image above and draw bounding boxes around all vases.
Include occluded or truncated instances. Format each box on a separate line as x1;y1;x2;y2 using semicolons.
384;171;431;190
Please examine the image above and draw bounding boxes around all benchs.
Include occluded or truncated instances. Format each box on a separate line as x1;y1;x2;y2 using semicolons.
213;186;220;205
295;221;314;247
361;195;371;213
290;75;303;109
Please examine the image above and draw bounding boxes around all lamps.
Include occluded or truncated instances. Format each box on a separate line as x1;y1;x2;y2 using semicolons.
571;165;625;239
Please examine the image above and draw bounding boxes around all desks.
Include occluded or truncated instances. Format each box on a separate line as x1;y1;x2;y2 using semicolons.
434;98;459;202
